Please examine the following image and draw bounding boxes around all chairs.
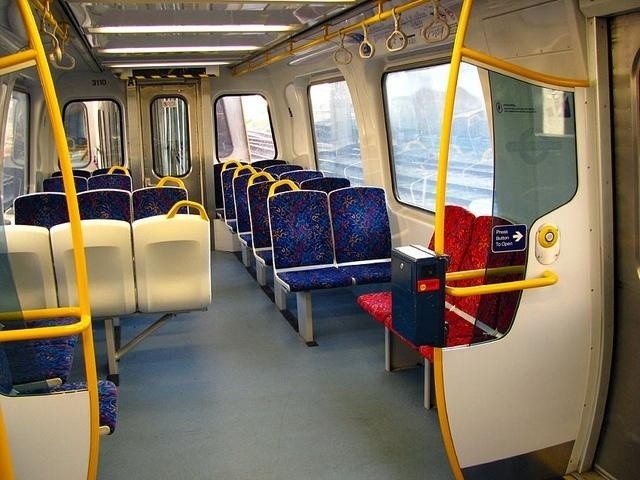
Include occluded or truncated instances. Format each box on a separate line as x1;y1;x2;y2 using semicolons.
214;158;391;347
1;317;118;435
0;164;210;386
356;205;527;409
